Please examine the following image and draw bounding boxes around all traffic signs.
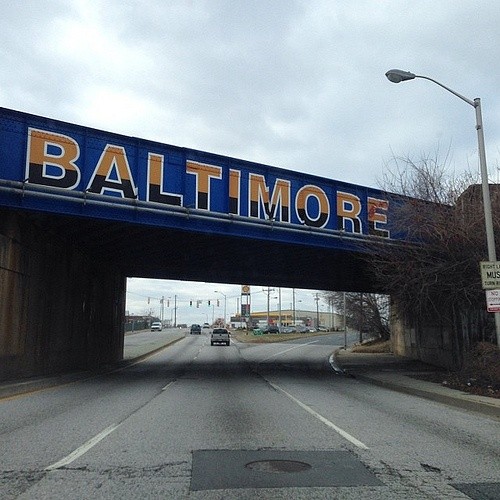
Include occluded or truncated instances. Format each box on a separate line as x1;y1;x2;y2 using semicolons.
486;289;500;313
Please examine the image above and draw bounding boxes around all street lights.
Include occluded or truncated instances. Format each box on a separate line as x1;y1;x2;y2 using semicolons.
317;304;325;331
293;300;302;325
214;290;227;328
267;296;277;326
384;68;500;349
160;297;171;320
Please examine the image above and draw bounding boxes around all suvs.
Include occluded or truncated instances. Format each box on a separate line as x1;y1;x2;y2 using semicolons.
190;324;202;335
209;329;231;346
151;322;162;332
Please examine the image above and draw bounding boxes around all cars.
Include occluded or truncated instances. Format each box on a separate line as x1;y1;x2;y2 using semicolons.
203;322;209;328
261;326;345;335
